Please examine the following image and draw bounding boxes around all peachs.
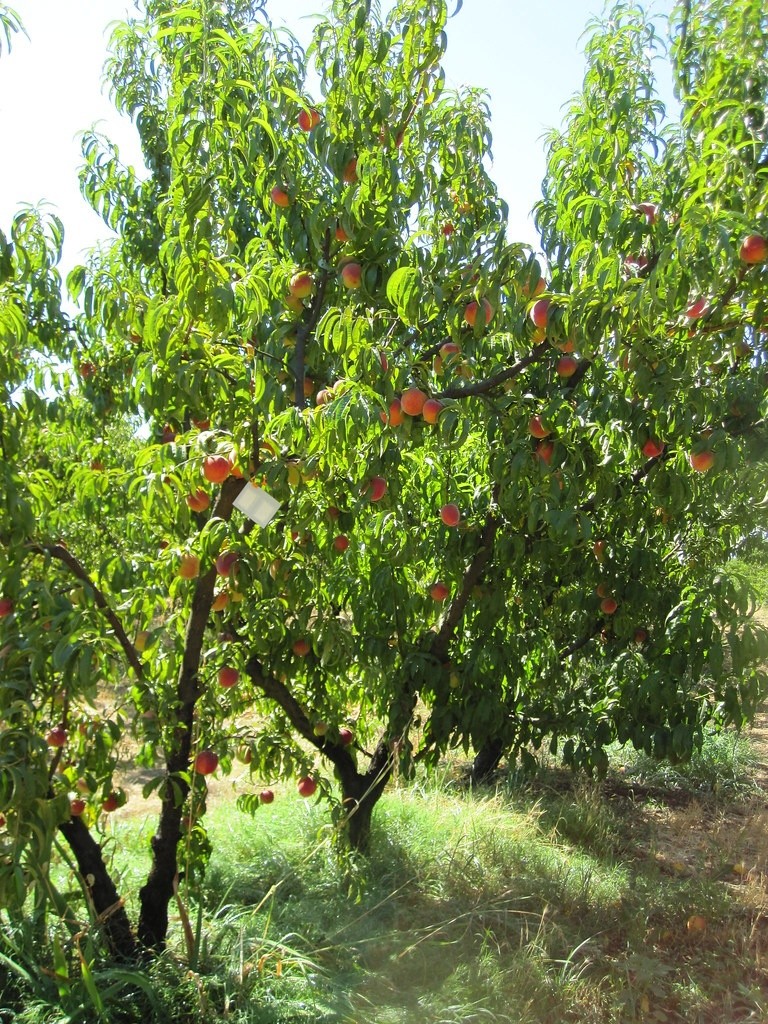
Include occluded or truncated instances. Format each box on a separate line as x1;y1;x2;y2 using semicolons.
0;108;768;934
